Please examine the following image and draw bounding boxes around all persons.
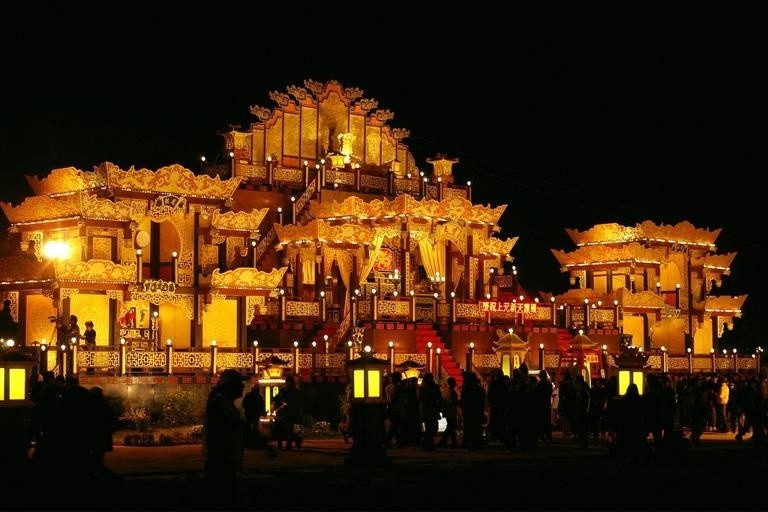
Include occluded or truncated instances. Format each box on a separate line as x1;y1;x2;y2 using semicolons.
28;367;112;467
338;364;764;455
621;336;629;347
205;369;273;512
242;385;265;433
70;315;79;344
566;321;578;335
79;321;96;346
